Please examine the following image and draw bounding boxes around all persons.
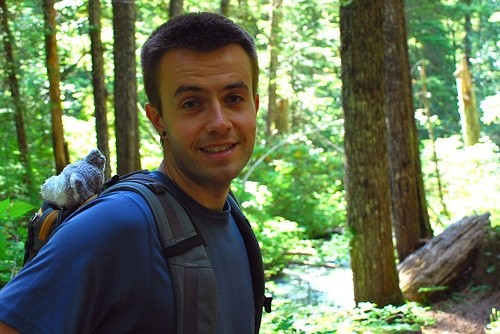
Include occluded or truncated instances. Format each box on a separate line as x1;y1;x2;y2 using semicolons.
0;12;272;334
